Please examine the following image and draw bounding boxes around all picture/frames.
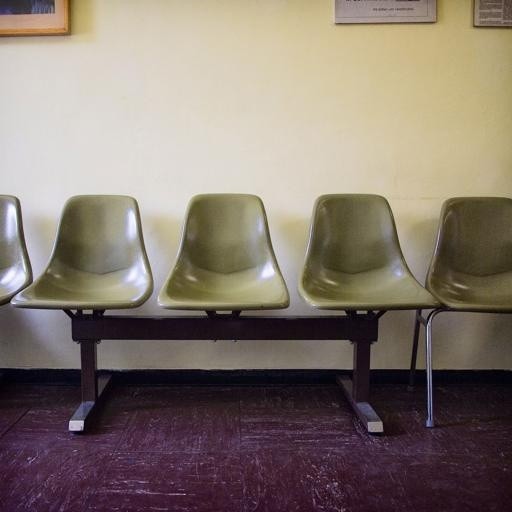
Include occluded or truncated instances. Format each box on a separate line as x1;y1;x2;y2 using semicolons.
472;1;511;30
0;1;69;37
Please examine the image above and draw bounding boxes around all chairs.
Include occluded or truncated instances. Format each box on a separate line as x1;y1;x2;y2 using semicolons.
0;193;512;436
